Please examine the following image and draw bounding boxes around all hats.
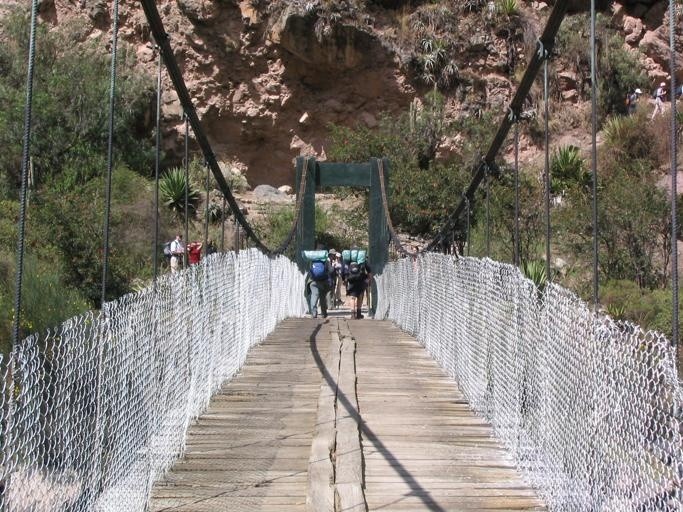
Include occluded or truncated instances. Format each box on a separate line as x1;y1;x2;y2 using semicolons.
327;248;337;255
335;253;341;258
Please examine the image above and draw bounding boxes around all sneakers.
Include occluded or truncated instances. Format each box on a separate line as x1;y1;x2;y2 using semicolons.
350;308;365;319
312;309;329;319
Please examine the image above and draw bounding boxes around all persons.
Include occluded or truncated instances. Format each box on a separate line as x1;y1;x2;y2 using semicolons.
171;233;217;274
626;88;642;118
303;249;371;320
651;82;666;122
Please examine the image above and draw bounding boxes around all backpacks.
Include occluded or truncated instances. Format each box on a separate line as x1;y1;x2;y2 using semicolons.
346;262;364;283
163;241;177;256
311;260;326;281
